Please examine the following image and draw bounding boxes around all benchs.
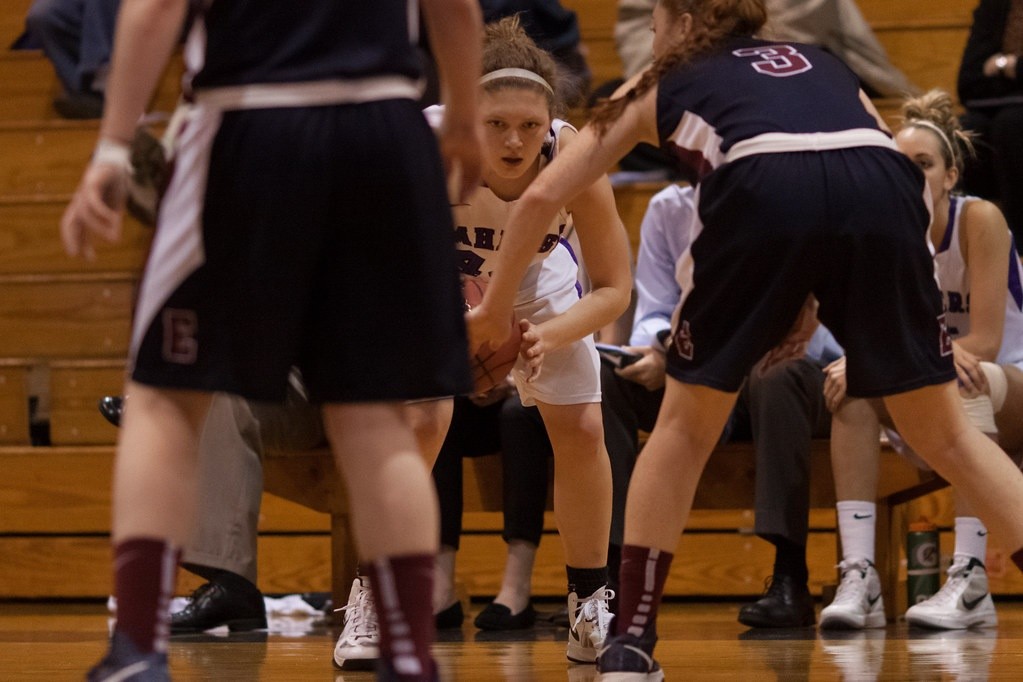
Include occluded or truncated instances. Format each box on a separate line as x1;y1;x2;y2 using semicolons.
0;0;1023;606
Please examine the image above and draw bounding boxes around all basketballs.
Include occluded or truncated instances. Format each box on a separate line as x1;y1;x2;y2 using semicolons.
453;273;522;399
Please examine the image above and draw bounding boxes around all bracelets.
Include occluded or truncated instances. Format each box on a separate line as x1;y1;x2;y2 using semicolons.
89;143;135;172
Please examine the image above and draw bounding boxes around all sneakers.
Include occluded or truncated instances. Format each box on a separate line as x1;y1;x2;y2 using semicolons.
818;553;887;628
565;581;616;662
904;557;998;629
380;660;438;682
595;616;665;682
333;576;383;669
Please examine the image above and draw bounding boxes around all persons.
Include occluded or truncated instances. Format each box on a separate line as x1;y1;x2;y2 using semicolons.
19;0;1023;682
58;1;487;682
455;1;1023;682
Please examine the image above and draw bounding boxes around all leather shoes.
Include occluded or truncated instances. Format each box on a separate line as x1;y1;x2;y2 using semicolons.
738;573;816;628
98;395;122;426
167;581;267;635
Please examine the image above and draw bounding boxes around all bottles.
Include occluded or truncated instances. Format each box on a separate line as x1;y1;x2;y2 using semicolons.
25;352;51;445
906;515;940;608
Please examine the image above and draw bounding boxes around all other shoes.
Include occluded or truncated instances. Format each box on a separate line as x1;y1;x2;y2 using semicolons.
85;634;168;682
434;600;464;628
473;597;533;630
